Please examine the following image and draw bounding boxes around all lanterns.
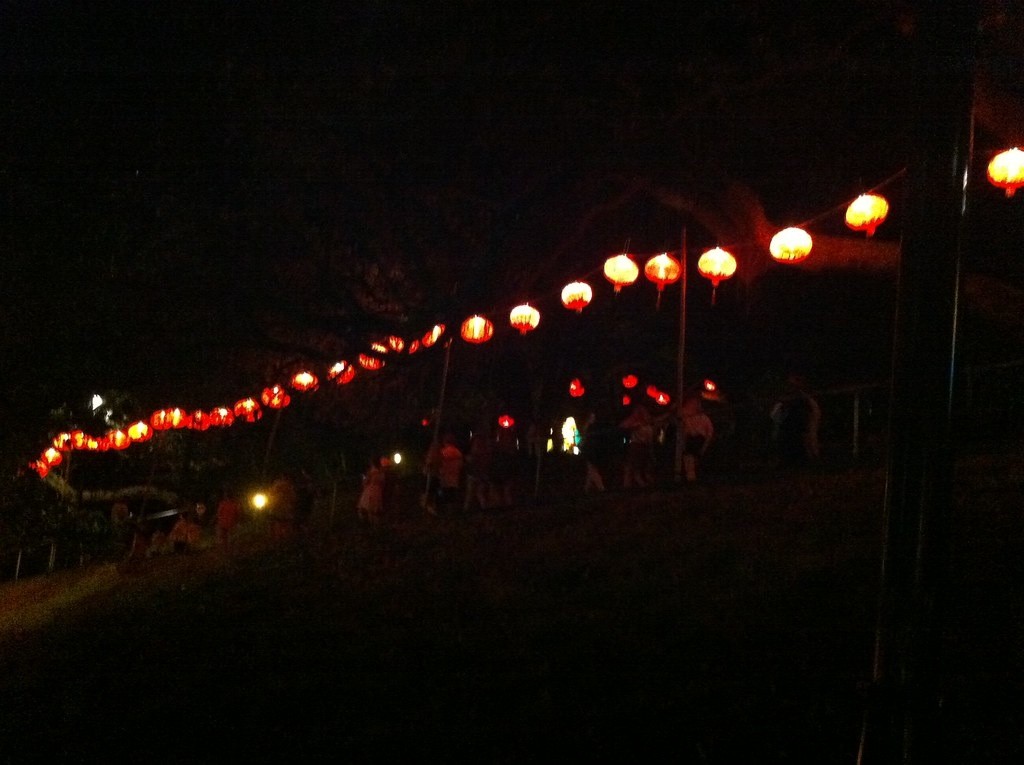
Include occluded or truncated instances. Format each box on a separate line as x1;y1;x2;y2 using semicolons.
28;137;1024;509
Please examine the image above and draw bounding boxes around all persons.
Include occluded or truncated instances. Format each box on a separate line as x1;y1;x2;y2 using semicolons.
143;368;823;556
